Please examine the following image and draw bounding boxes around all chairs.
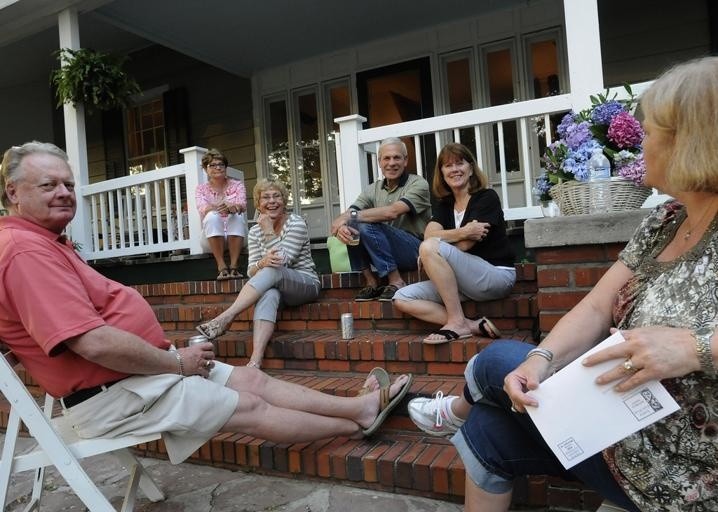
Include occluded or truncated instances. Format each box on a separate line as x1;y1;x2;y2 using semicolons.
0;344;167;511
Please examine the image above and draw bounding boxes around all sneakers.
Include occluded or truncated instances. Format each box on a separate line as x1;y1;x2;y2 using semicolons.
216;268;243;279
354;280;406;301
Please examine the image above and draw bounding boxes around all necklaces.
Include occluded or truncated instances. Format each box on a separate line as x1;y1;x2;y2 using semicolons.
675;200;716;241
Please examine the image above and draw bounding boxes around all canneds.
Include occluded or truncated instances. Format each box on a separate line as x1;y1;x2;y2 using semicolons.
188;336;209;379
341;314;354;340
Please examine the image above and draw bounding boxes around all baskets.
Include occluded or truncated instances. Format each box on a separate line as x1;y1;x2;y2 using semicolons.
548;181;653;215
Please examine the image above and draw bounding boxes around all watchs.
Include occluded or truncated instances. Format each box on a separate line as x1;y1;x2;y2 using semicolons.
692;322;717;378
349;209;359;222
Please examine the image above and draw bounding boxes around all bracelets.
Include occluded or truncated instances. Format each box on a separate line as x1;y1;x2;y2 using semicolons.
255;260;263;271
235;204;241;215
172;348;186;375
522;345;557;377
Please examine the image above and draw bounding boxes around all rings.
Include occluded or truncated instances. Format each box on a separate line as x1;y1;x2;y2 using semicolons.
624;357;639;374
205;359;213;369
509;402;519;415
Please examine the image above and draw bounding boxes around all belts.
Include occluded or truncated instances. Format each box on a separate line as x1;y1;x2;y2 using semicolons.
59;383;118;408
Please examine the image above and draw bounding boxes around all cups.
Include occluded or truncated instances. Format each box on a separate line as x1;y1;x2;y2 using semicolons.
539;199;560;218
345;219;361;246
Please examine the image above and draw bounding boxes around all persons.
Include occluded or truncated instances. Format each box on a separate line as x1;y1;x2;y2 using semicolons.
330;136;437;304
0;141;417;468
406;53;717;511
192;148;250;284
192;178;324;368
392;141;520;346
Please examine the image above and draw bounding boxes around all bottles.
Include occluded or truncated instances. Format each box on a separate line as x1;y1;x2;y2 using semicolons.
585;147;612;216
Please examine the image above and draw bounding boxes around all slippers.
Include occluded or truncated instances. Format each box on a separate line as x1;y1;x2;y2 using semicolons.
423;329;473;344
478;317;500;338
357;367;461;436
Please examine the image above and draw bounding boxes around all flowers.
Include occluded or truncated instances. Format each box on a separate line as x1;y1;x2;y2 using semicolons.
516;83;645;208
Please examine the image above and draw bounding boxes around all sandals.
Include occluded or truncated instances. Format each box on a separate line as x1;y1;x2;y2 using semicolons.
196;320;225;340
